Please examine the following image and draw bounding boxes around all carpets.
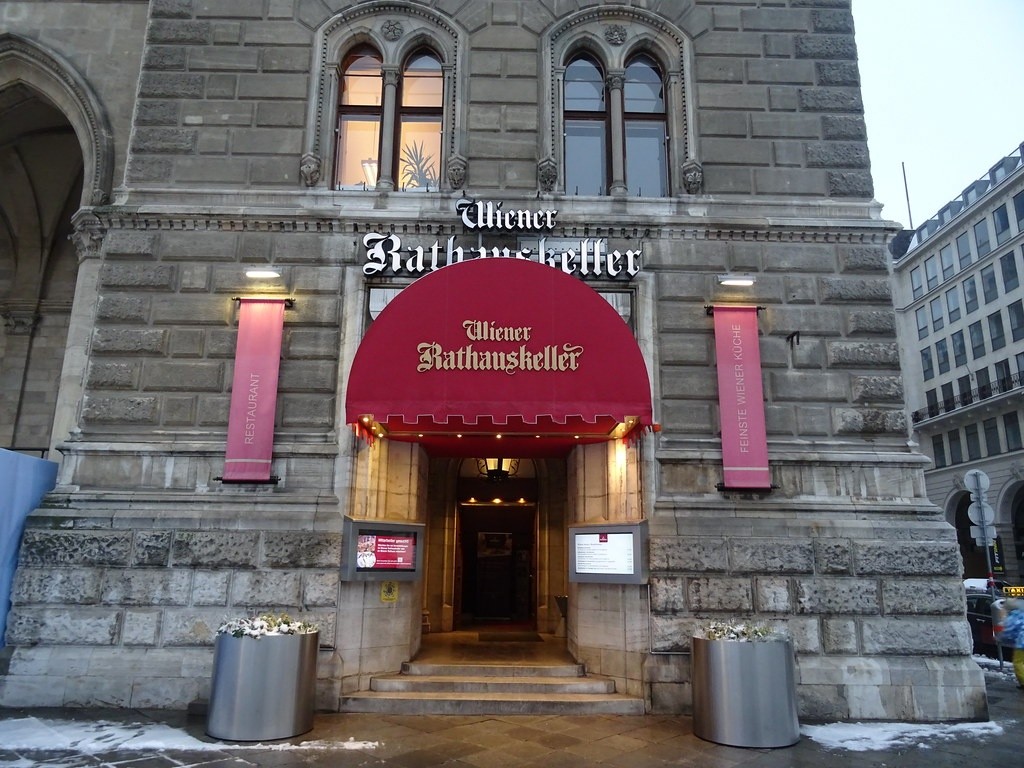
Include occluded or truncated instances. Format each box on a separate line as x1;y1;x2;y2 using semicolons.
477;631;544;642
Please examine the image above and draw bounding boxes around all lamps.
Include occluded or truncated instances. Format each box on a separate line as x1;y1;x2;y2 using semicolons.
476;457;520;483
362;159;378;185
242;264;282;278
715;272;756;287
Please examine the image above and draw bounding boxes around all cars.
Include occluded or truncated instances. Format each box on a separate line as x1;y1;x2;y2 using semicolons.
966;587;1024;659
963;577;1012;592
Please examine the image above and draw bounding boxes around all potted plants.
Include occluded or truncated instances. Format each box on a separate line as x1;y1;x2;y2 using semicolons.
206;614;319;741
693;618;801;748
